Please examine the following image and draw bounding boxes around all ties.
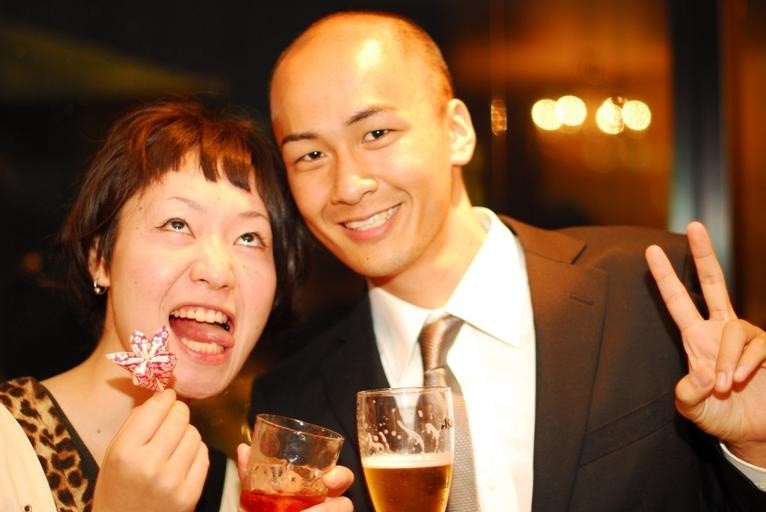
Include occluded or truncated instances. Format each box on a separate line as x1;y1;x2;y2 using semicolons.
413;313;482;511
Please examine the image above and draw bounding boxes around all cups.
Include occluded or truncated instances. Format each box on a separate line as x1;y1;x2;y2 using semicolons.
239;386;454;512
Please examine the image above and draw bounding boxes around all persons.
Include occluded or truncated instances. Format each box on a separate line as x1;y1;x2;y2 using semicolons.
1;98;356;512
250;10;765;511
